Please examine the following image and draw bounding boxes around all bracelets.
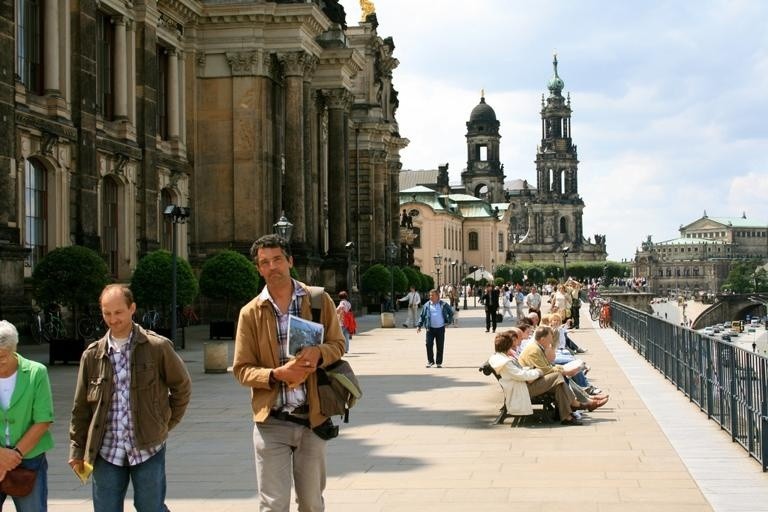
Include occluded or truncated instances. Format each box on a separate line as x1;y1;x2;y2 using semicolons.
14;447;22;457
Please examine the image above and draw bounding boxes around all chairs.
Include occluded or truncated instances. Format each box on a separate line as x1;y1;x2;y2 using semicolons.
479;360;558;427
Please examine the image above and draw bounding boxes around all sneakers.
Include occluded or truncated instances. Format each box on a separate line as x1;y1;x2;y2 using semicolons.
437;363;442;367
426;361;435;368
561;385;609;425
578;348;587;353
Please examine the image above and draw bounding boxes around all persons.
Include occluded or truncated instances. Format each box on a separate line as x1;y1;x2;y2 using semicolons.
335;291;352;354
397;285;421;328
416;289;454;368
752;342;756;352
438;276;647;425
231;235;347;512
67;283;192;512
0;319;55;512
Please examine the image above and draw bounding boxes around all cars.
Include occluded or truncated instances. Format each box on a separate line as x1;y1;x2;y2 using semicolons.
702;315;760;341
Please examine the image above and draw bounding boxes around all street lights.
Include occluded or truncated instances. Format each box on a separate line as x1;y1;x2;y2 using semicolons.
273;209;293;245
461;262;484;310
450;261;456;287
562;247;569;282
385;242;397;311
433;253;442;292
345;241;355;299
164;204;190;347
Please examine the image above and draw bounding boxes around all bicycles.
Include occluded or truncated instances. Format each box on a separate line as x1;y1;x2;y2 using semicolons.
589;298;612;328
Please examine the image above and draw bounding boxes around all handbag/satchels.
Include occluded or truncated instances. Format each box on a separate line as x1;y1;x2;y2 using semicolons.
572;298;581;308
510;294;513;301
566;308;572;318
1;466;37;497
317;360;363;416
496;314;502;322
